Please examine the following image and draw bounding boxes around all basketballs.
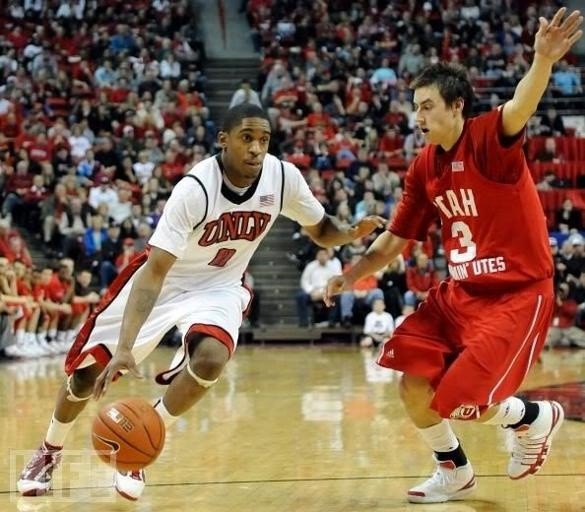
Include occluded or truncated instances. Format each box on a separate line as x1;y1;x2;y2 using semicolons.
92;396;165;472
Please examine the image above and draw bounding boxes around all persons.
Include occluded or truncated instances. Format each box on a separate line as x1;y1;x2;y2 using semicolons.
5;353;68;456
359;353;396;397
320;6;585;503
179;356;253;439
0;1;585;361
339;364;380;456
16;102;387;501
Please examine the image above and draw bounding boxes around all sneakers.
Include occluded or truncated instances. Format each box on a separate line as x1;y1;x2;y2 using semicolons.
407;454;477;503
15;441;63;496
506;400;565;480
112;468;146;501
